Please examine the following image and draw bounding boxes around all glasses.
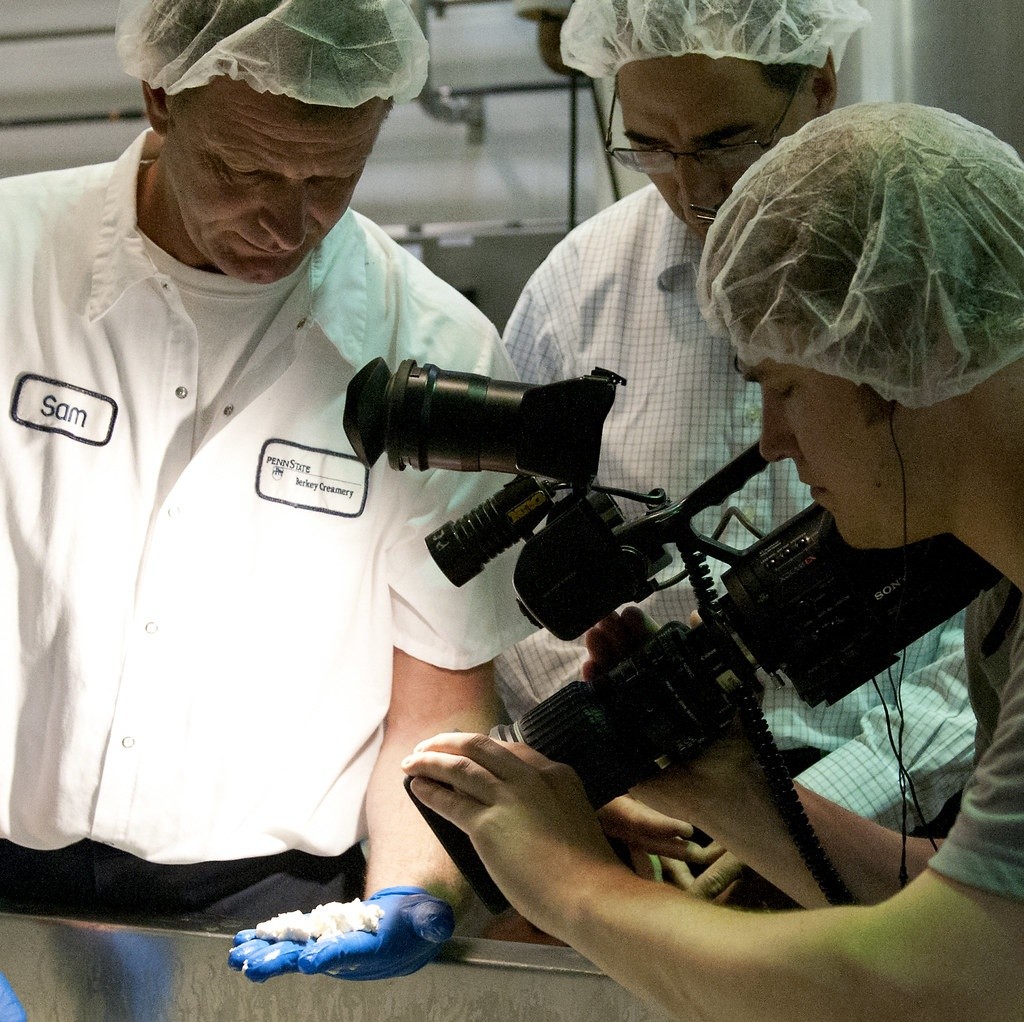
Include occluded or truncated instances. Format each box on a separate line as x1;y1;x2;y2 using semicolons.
601;71;808;174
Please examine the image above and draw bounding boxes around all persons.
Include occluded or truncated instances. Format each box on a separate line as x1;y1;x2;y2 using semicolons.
499;0;977;911
402;99;1024;1019
1;1;523;1019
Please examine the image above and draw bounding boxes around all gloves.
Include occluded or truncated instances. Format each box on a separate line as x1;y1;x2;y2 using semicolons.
227;884;454;984
